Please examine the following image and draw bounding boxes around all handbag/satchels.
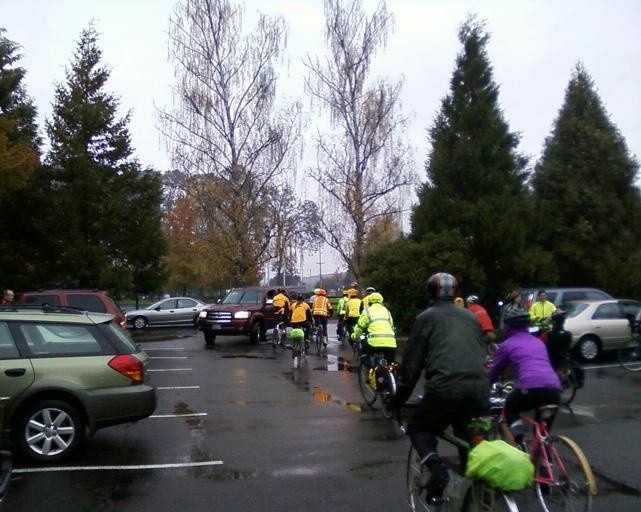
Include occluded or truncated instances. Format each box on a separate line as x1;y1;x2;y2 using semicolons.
369;366;390;391
290;329;304;339
466;441;533;490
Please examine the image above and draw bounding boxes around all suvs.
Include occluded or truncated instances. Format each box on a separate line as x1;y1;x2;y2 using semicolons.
12;289;128;330
0;306;158;465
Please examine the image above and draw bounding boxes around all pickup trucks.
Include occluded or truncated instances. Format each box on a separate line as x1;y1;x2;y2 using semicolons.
195;286;314;346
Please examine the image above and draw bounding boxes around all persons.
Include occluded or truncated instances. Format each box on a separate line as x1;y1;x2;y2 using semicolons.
383;272;491;504
345;288;365;343
488;312;565;494
0;289;14;305
350;292;398;389
288;296;313;343
310;288;333;347
336;290;349;340
499;289;571;372
272;289;290;337
466;294;495;333
359;287;376;315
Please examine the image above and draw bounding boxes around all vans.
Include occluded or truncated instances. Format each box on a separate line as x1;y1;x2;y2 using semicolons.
502;289;617;332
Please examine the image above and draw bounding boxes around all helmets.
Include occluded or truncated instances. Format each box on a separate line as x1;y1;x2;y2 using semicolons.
278;282;384;303
427;273;457;299
467;296;478;303
503;309;530;328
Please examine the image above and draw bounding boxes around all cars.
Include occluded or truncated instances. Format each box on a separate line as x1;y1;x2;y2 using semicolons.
549;299;641;363
124;296;216;330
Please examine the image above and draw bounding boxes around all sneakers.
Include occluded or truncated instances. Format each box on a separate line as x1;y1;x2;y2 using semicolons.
426;470;450;503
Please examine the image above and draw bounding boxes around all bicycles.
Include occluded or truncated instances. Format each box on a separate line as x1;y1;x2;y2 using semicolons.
350;335;396;420
618;318;640;372
550;359;577;405
311;314;335;353
271;316;290;354
490;380;599;511
288;310;315;385
392;395;521;512
335;311;362;357
482;331;501;362
